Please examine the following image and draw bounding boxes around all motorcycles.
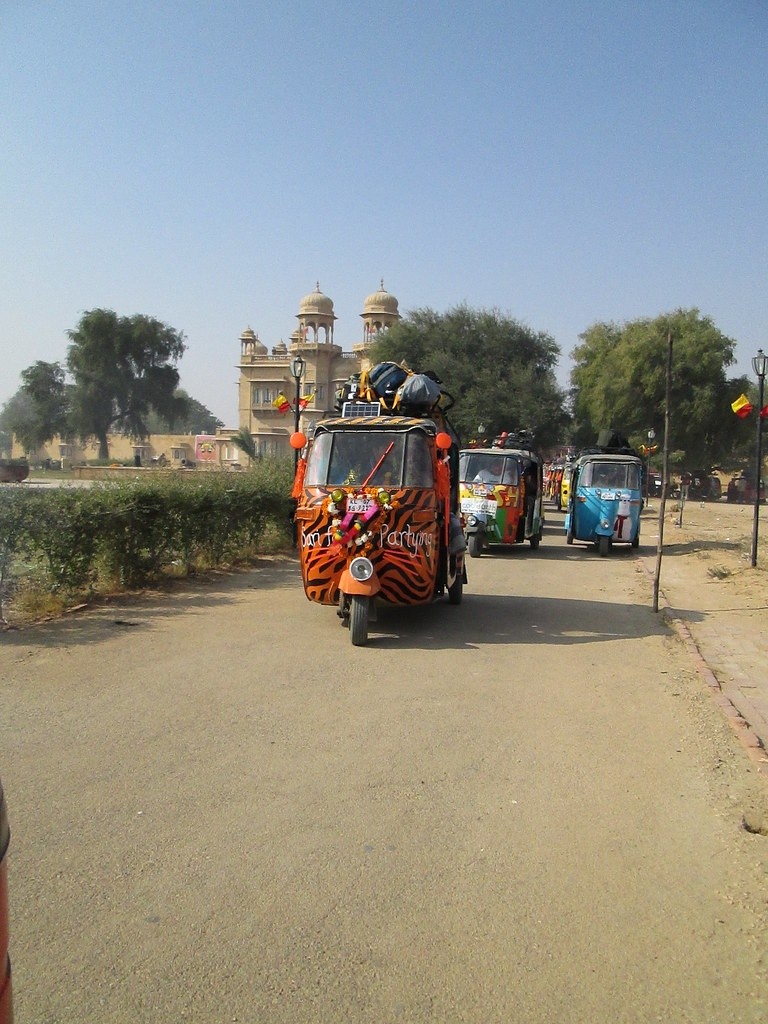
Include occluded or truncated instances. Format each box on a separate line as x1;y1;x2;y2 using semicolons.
291;415;468;646
455;449;545;559
566;455;646;558
542;460;573;513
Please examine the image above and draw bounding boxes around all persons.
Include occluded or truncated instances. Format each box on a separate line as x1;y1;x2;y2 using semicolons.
346;435;400;485
473;459;512;484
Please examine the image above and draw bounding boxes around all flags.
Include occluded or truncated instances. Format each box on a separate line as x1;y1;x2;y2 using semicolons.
731;393;753;419
293;395;312;409
272;396;289;413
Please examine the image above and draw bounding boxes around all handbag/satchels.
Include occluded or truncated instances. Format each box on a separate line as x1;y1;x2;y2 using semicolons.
340;360;445;410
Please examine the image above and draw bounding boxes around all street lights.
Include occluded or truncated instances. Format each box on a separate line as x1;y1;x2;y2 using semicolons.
478;420;485;449
646;428;657;507
751;347;768;566
290;352;306;551
